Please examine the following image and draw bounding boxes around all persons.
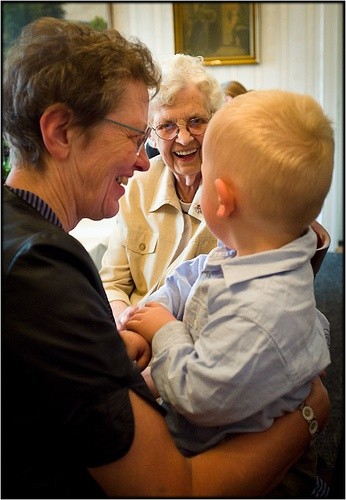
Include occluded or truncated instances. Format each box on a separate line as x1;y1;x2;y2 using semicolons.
222;81;246;99
122;90;337;457
0;17;329;500
99;53;331;318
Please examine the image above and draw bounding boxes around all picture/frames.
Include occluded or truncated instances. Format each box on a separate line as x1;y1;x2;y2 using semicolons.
172;3;260;65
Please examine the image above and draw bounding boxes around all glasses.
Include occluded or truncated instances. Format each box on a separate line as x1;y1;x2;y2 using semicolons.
92;116;152;157
149;111;213;140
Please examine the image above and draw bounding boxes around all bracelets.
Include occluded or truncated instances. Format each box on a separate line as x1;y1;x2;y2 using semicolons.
299;403;319;446
